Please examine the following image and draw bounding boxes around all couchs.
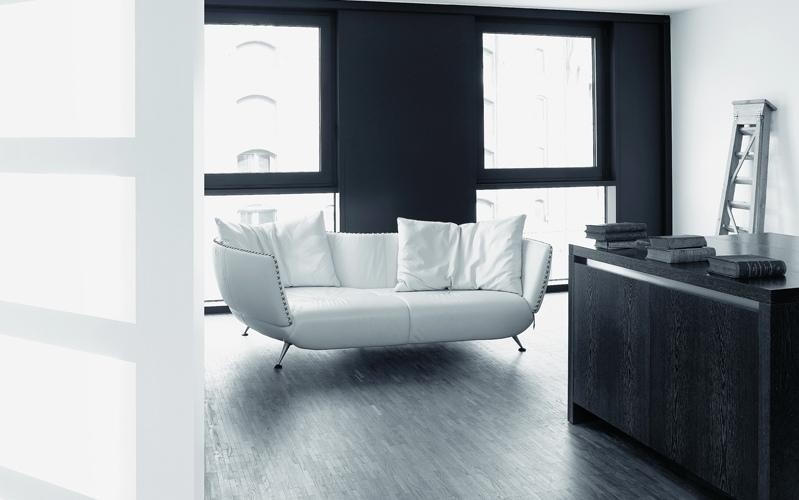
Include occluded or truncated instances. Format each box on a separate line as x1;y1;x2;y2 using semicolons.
209;230;554;370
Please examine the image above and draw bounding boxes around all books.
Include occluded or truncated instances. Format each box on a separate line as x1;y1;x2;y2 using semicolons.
646;247;717;264
584;222;650;250
706;254;786;279
647;234;706;249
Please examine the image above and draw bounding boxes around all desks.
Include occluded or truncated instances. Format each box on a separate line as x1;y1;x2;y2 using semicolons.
565;233;799;497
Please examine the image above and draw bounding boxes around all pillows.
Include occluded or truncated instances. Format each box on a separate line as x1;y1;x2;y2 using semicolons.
212;209;344;292
390;212;527;298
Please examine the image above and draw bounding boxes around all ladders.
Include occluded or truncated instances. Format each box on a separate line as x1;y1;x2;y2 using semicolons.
715;98;777;235
